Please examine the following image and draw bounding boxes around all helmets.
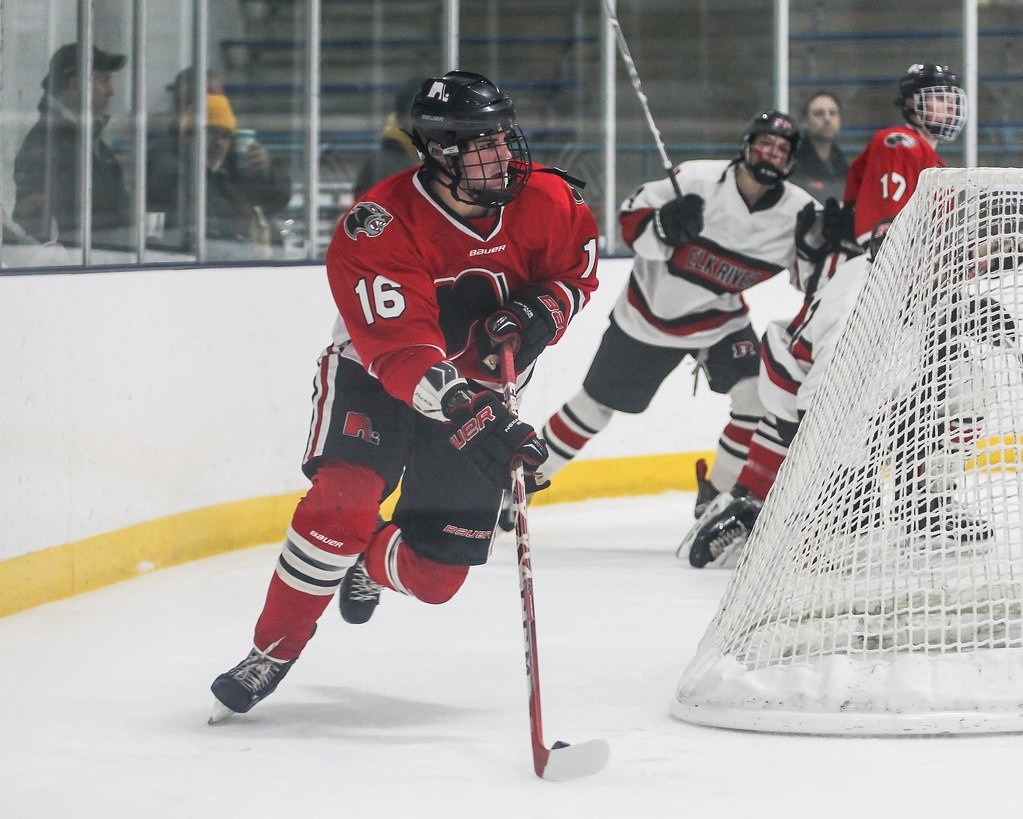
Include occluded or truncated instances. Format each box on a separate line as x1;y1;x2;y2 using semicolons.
742;109;800;184
399;68;516;147
866;217;914;270
895;61;961;118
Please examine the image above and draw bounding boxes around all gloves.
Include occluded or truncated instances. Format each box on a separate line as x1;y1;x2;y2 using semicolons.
445;391;552;495
795;197;842;262
653;192;706;247
478;286;566;377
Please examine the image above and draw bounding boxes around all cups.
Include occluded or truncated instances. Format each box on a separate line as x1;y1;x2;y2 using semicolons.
233;129;256;170
144;210;166;240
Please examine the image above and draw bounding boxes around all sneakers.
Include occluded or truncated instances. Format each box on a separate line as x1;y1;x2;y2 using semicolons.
206;623;317;724
675;491;764;569
339;509;392;625
694;458;750;518
498;490;534;532
895;478;995;557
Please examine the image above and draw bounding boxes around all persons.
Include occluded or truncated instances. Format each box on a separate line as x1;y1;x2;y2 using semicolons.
352;78;425;200
11;46;295;247
499;64;1023;569
207;71;600;725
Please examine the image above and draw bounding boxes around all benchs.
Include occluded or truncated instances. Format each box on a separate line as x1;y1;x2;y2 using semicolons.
788;31;1023;137
109;31;601;160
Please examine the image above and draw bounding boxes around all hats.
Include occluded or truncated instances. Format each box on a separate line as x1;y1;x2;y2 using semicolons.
41;43;128;91
180;94;236;138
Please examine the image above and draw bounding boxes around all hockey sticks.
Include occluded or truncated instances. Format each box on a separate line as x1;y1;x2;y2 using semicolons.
829;228;865;254
495;340;610;782
605;1;686;202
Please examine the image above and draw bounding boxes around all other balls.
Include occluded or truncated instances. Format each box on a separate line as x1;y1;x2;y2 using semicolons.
549;742;570;750
